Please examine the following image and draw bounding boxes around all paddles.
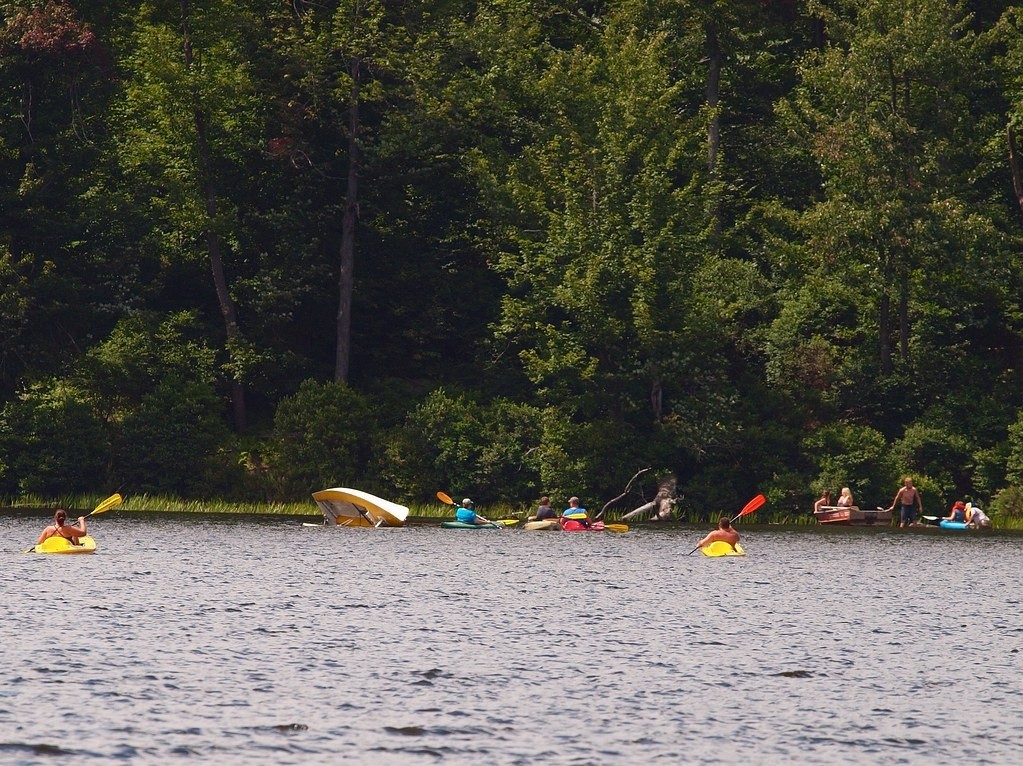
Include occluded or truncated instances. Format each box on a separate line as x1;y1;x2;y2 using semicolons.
437;492;503;530
923;515;947;521
497;513;587;525
688;494;766;556
25;493;122;553
528;516;629;533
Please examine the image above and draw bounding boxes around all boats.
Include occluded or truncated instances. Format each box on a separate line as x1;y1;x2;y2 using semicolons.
34;531;97;555
700;540;746;556
939;519;971;531
562;519;606;532
310;486;411;531
440;519;519;529
525;515;560;530
814;506;866;525
850;507;893;526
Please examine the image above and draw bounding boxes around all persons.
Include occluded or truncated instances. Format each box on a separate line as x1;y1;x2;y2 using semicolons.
561;496;592;524
939;500;992;529
536;496;557;520
697;517;740;550
813;489;830;514
889;478;922;527
456;498;490;525
837;487;853;507
37;510;86;545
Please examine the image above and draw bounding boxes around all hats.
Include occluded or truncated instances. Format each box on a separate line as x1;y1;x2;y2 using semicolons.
568;497;579;504
463;498;473;504
